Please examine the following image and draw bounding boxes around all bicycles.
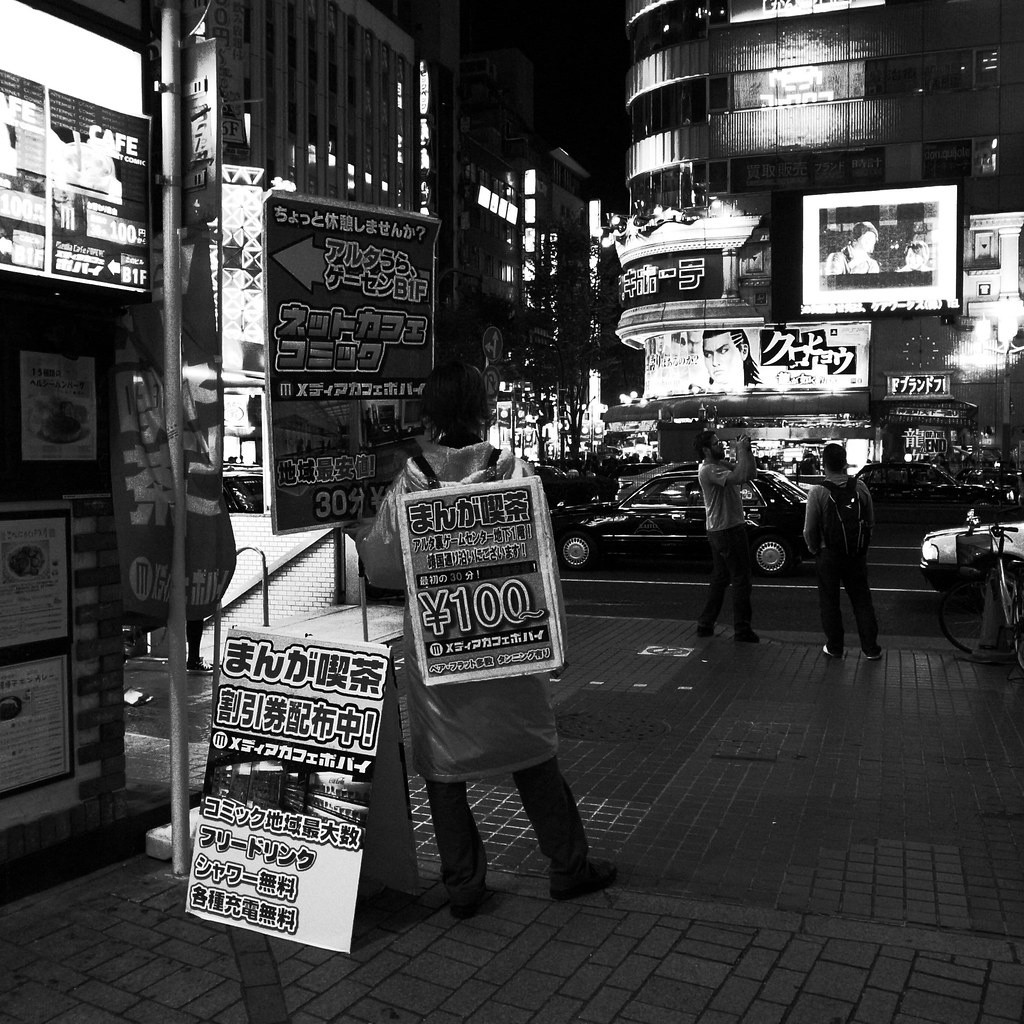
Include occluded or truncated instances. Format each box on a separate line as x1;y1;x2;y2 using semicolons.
938;498;1024;679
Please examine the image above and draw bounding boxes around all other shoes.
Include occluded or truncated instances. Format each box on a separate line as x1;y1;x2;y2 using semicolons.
867;645;883;659
549;859;617;902
451;885;486;920
697;625;714;637
734;632;759;643
822;645;841;657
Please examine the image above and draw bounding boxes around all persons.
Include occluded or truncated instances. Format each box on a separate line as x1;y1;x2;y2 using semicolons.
703;330;762;390
898;242;930;271
358;363;616;918
824;222;879;274
693;431;759;643
803;444;883;659
187;618;220;675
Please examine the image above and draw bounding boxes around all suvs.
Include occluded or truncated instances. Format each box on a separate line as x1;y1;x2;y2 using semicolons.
856;461;994;515
954;467;1021;506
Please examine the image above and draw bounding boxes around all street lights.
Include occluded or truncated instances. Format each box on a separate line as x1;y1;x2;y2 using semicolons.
501;410;525;454
996;313;1017;470
517;414;534;461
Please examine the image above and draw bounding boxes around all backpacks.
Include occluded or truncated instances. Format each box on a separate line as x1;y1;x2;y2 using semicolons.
823;478;869;556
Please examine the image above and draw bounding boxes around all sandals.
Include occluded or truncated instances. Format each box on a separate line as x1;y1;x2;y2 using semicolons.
123;684;154;707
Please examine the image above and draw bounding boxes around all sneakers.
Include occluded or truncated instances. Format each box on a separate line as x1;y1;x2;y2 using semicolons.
187;658;214;676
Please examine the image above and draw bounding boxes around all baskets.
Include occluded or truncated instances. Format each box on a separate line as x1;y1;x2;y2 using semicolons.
957;535;992;577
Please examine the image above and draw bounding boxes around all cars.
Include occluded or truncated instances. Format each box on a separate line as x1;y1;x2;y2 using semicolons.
223;471;266;515
918;520;1024;597
615;460;702;504
550;468;825;579
